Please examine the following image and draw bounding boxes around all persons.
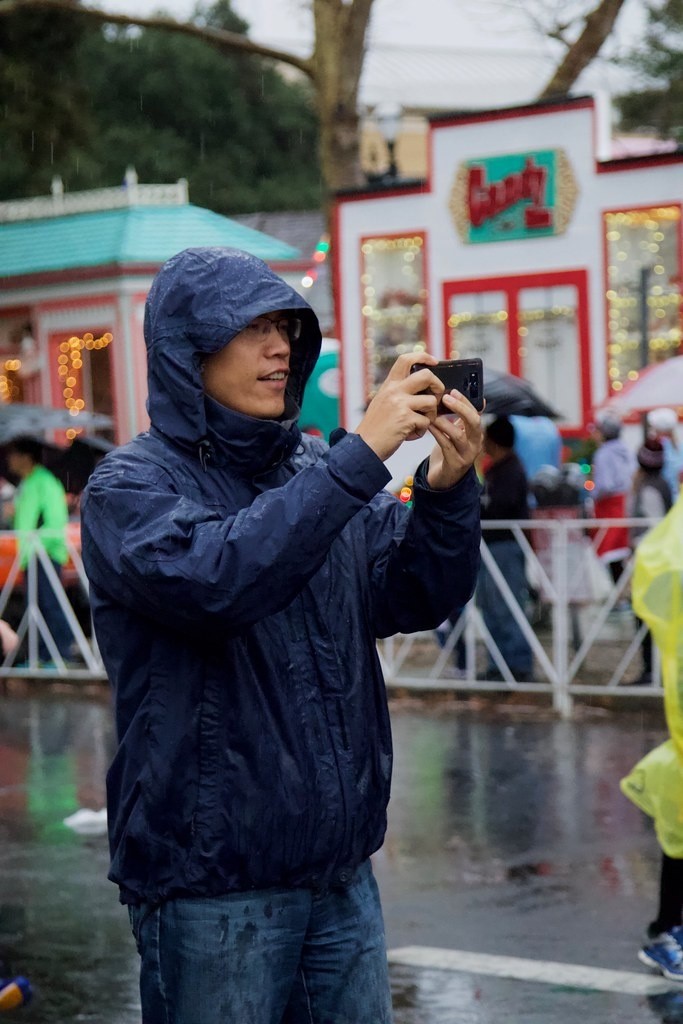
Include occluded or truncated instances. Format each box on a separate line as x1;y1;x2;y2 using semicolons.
0;436;75;659
620;483;682;980
588;405;683;685
78;246;487;1024
453;414;563;681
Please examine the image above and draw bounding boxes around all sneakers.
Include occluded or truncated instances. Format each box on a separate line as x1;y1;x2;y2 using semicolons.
636;919;683;980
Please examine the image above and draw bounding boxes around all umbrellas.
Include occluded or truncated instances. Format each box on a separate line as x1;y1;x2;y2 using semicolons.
608;355;683;421
483;367;559;419
0;432;116;495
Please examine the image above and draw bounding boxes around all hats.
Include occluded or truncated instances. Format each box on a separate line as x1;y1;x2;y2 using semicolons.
638;440;665;467
647;408;677;433
593;411;620;437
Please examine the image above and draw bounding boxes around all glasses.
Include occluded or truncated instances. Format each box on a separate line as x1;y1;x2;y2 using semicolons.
243;315;302;341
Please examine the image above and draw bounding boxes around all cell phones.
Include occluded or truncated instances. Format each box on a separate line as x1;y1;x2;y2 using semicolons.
411;359;485;415
